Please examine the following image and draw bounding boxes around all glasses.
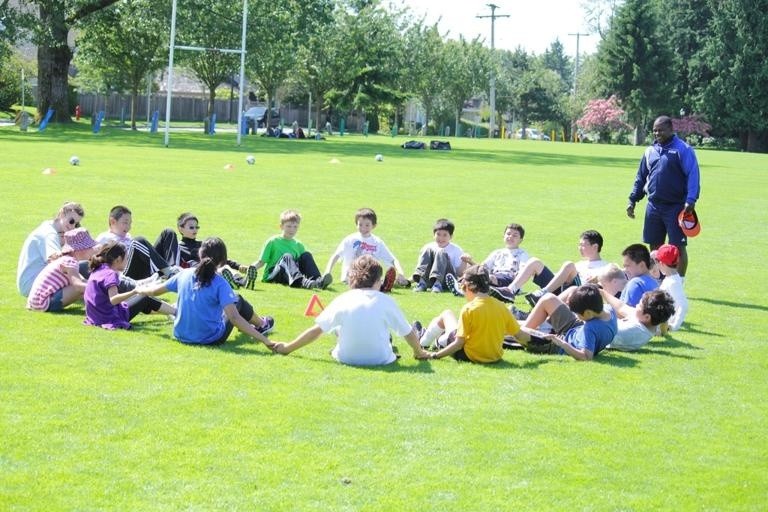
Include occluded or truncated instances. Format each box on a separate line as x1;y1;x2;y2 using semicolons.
69;219;81;228
190;226;200;229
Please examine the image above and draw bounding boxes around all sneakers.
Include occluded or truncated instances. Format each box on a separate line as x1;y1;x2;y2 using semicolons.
222;266;332;290
254;316;274;334
380;267;543;350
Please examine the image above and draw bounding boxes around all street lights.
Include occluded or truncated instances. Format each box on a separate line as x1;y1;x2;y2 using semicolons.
679;104;690;118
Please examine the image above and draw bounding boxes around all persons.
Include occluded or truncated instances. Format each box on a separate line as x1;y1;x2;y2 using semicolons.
578;132;585;143
627;114;702;287
17;201;276;353
248;208;689;366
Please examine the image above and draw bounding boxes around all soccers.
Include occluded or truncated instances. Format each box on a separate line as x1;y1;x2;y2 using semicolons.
245;156;255;165
68;157;80;166
375;153;384;162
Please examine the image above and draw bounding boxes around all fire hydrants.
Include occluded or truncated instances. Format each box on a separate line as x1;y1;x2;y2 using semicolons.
74;105;80;120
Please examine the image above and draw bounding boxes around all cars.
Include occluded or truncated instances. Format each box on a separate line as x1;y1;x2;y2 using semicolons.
514;128;551;141
243;106;281;129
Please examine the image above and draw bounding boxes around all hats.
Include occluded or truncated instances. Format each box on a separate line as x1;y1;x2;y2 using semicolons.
62;227;99;254
650;244;680;266
678;209;700;237
